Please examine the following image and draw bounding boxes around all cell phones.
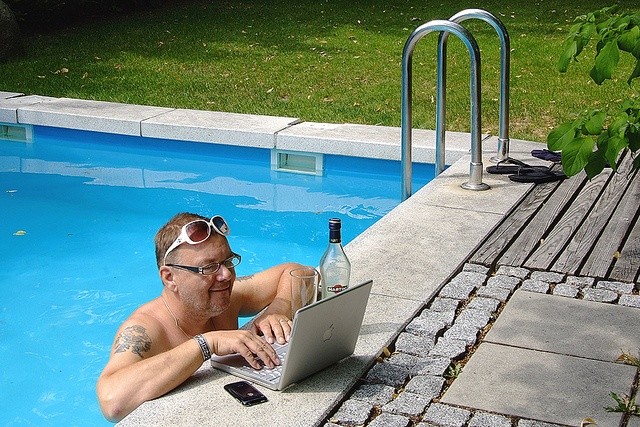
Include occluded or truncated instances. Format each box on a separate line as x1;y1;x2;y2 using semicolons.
224;381;267;407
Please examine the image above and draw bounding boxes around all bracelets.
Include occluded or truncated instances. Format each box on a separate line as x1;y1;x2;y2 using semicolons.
193;333;212;360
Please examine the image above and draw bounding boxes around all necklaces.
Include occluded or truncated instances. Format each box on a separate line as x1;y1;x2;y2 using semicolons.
161;295;216;340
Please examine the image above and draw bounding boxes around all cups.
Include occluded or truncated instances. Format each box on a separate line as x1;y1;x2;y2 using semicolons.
290;268;318;322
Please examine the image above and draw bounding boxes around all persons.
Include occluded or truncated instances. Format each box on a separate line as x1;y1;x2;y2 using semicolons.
97;213;315;424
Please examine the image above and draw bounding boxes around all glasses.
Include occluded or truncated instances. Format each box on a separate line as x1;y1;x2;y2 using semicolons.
164;215;231;267
166;251;240;275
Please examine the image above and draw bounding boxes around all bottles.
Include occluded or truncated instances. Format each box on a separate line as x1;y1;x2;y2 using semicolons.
319;217;351;299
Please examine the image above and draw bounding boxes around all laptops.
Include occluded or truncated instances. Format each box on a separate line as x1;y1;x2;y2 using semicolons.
210;279;373;392
